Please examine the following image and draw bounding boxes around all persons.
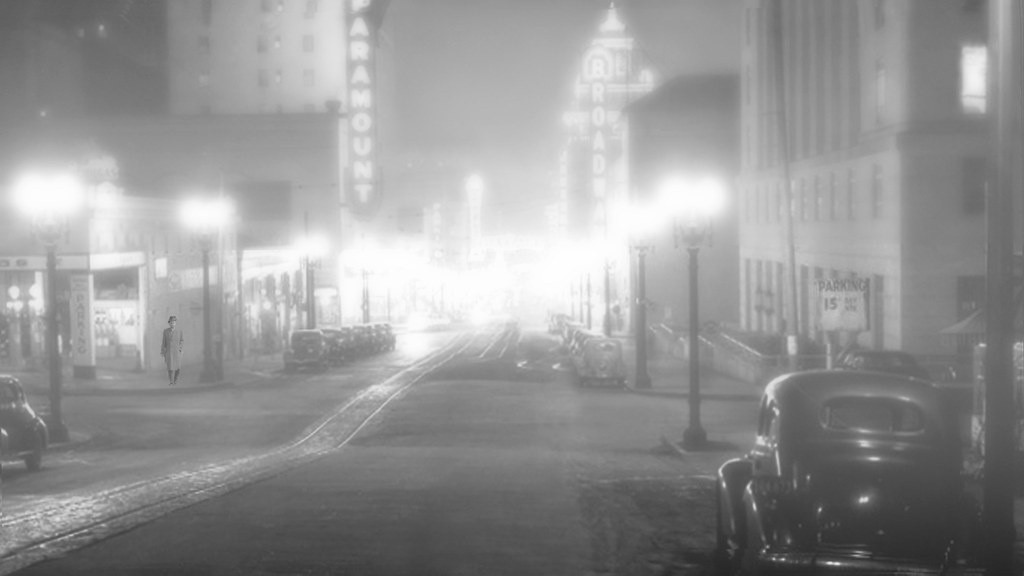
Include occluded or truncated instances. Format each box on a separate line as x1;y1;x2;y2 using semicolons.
160;316;184;387
836;340;867;369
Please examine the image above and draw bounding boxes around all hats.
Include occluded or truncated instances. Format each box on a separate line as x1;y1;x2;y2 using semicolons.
167;316;178;322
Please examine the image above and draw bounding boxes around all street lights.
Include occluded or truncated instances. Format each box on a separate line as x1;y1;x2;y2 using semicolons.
3;164;96;419
617;196;674;388
656;168;732;453
175;191;238;366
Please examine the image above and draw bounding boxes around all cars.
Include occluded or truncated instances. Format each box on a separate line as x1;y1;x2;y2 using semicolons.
840;349;933;380
0;374;48;473
718;368;994;576
282;323;395;370
547;309;627;387
